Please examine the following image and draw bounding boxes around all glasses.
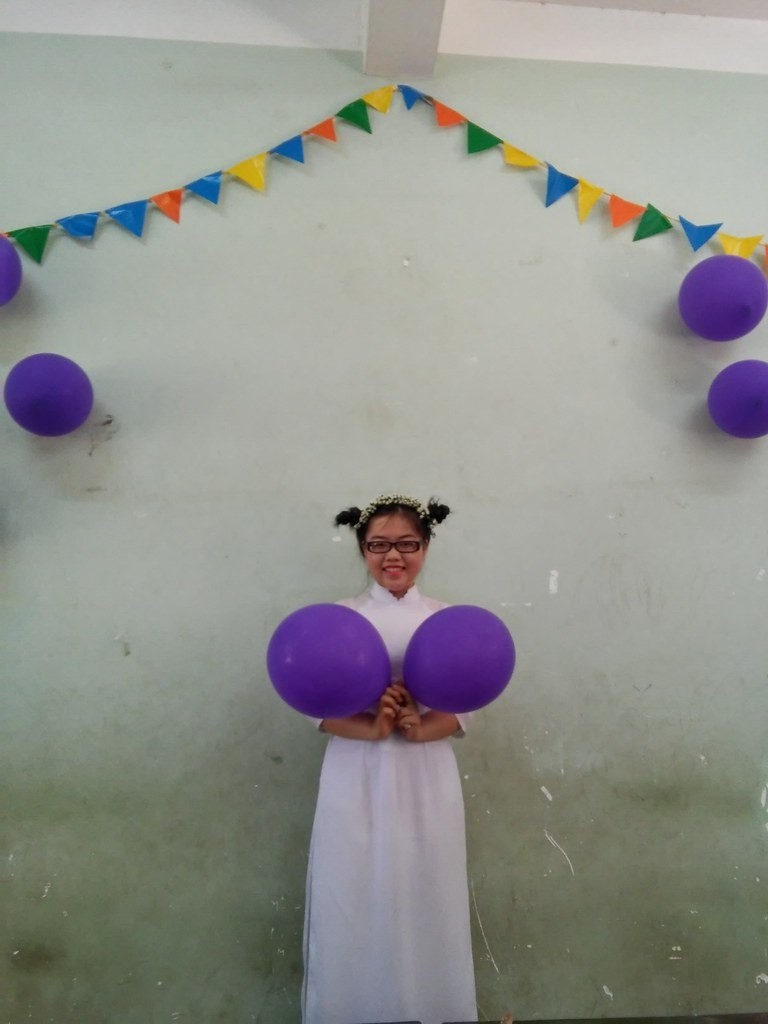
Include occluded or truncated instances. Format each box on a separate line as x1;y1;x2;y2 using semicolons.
361;540;424;553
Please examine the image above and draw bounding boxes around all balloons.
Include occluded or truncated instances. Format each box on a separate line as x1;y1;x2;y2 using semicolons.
402;604;515;713
3;353;94;437
677;254;768;342
0;234;22;308
709;359;768;442
266;604;392;719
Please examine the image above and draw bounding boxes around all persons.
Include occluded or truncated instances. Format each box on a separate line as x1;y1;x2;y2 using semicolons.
301;494;477;1024
